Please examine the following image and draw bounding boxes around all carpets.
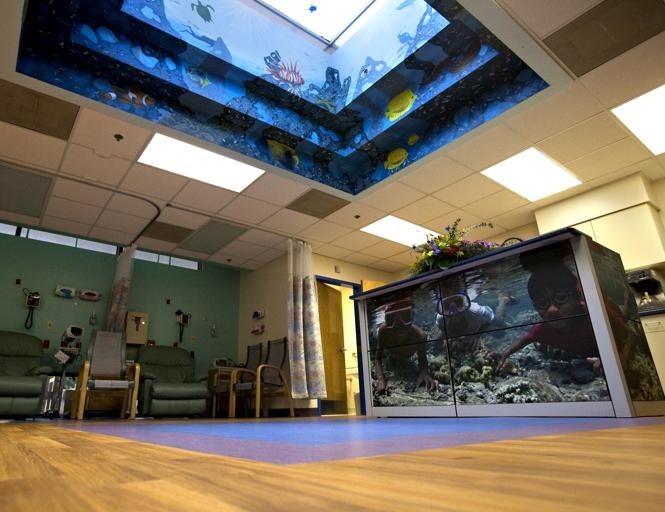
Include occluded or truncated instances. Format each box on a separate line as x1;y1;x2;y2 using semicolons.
59;417;663;467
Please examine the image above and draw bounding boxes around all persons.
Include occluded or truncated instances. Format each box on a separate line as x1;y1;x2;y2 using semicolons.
487;262;604;378
375;298;439;395
435;277;520;346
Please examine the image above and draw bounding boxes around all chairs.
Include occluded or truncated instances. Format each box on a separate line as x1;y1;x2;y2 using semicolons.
1;331;54;422
137;345;208;418
208;337;294;419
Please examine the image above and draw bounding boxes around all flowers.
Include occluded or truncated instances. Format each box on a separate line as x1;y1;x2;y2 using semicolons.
407;217;499;274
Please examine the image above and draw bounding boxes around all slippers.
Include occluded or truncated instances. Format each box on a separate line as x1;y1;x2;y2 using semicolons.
503;295;519;305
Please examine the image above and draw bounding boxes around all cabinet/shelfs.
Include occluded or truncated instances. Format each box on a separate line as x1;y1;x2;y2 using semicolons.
641;314;664;331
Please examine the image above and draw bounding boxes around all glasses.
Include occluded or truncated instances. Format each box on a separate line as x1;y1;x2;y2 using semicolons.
533;287;574;310
437;291;471;316
384;306;412;328
